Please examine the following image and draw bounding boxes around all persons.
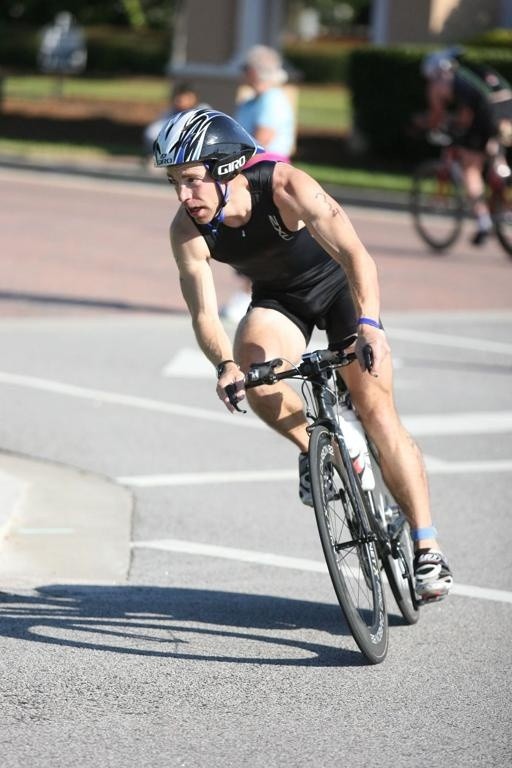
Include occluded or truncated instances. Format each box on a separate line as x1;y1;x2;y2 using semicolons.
153;105;454;596
231;43;299;163
411;49;512;248
142;85;215;174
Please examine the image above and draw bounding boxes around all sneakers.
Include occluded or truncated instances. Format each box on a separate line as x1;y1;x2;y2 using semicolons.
474;230;488;245
298;445;336;507
413;547;453;595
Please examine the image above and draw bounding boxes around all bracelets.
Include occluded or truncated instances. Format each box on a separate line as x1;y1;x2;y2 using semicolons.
357;317;383;332
216;358;237;378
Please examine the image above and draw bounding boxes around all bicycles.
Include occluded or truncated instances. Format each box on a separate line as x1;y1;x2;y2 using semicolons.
411;126;512;254
226;343;446;662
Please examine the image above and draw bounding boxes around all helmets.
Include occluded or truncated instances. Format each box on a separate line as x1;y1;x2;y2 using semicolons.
422;50;462;80
152;107;257;184
